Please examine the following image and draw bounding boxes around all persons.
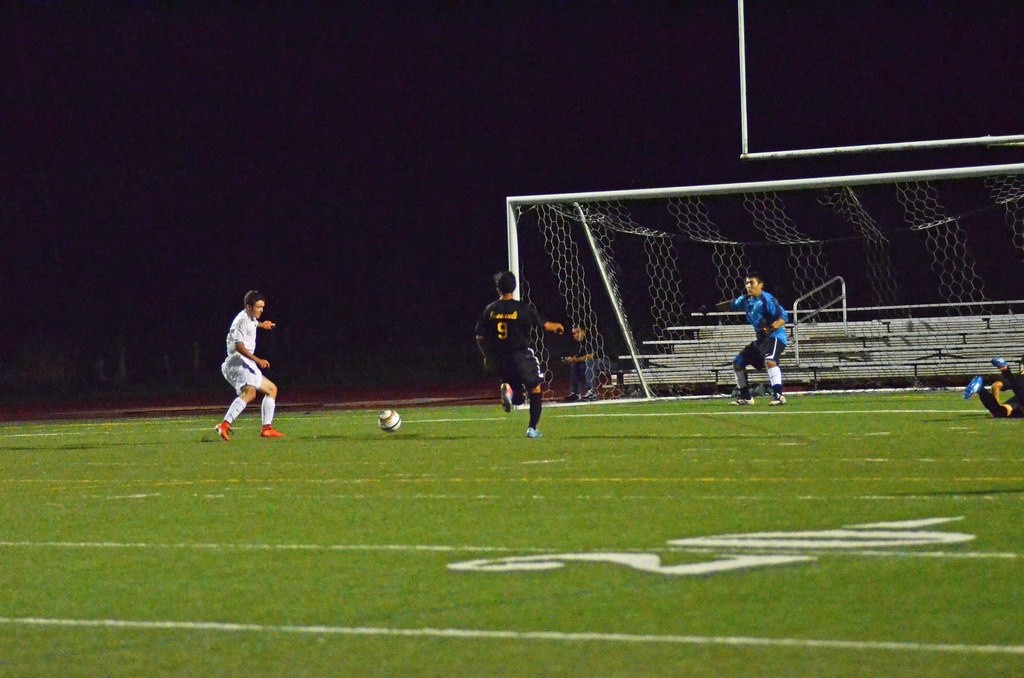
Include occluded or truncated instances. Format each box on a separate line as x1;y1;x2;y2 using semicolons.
558;323;622;403
697;272;788;406
475;272;564;438
963;355;1024;419
215;290;286;441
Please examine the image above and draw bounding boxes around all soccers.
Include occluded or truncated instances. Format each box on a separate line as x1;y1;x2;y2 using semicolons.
376;408;403;433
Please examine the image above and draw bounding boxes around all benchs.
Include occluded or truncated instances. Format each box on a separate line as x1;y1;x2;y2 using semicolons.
604;299;1024;395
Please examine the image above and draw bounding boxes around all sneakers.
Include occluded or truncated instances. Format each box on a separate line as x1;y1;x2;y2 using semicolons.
526;427;544;438
962;375;983;400
768;395;787;406
214;422;234;441
500;382;514;414
581;388;598;401
261;427;285;437
990;356;1008;368
564;391;583;402
728;397;755;406
